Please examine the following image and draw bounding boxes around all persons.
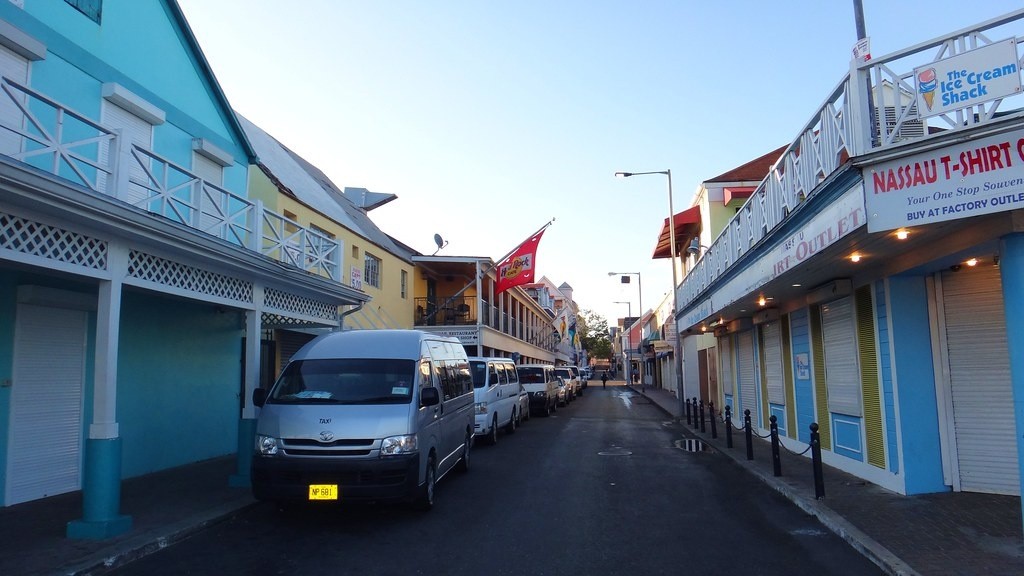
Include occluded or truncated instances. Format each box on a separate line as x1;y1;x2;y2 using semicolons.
633;367;639;383
601;371;607;388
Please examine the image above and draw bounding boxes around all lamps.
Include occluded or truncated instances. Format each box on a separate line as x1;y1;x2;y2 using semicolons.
687;237;709;255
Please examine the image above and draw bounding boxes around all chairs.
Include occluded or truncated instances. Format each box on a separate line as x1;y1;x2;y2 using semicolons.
448;304;471;324
416;303;435;325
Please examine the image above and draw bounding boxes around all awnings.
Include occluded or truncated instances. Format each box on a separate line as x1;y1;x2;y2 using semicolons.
651;205;701;259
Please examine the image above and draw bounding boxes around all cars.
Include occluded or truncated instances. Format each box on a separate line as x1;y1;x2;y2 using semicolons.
554;363;592;400
555;375;570;408
517;382;530;426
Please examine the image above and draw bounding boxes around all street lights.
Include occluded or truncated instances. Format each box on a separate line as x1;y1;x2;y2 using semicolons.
608;271;646;394
614;168;686;419
613;301;634;386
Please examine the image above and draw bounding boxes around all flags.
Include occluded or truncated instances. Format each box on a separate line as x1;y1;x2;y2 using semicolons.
553;309;568;341
569;322;579;346
494;227;547;301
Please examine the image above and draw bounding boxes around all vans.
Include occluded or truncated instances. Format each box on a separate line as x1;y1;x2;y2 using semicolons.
514;363;559;417
464;355;522;444
248;328;478;509
